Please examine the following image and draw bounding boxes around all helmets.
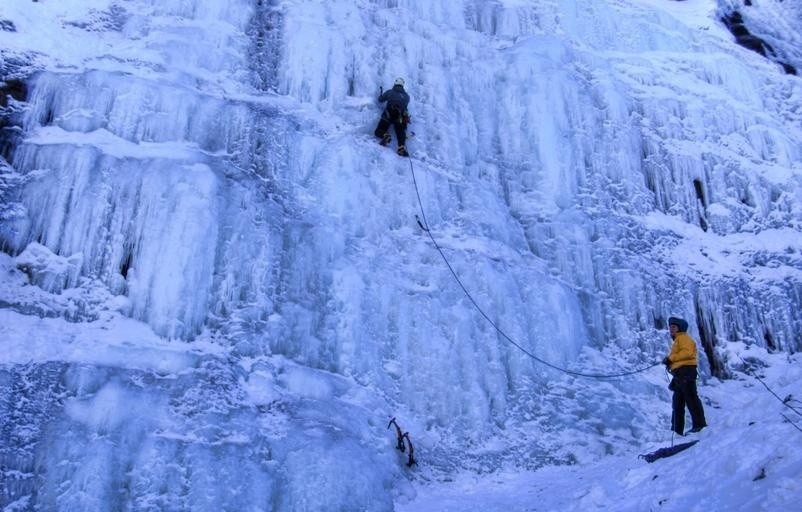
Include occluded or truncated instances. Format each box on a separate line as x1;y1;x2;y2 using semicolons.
393;76;405;87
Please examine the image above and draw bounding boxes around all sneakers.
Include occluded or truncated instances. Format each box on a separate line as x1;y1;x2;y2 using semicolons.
397;145;410;156
378;133;392;146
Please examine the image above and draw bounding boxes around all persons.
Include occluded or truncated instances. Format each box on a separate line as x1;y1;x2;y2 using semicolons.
661;317;708;436
375;77;411;157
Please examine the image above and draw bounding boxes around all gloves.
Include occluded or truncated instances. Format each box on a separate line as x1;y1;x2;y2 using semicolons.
661;356;673;368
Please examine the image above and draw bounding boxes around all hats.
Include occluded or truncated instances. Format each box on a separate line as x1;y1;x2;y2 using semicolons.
668;316;689;334
